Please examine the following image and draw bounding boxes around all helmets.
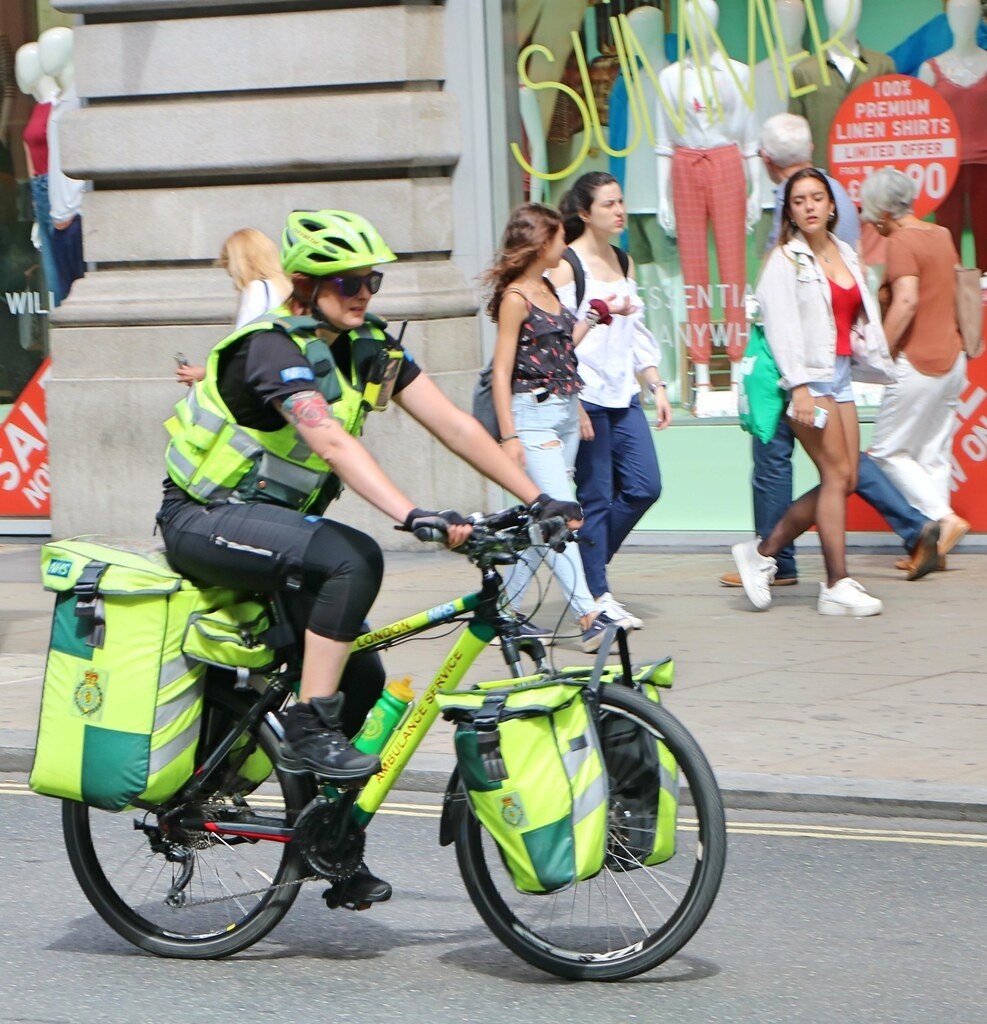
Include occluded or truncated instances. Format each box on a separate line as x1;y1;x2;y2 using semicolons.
280;208;398;279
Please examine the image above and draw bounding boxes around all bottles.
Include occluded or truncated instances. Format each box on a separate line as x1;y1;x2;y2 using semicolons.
353;675;414;758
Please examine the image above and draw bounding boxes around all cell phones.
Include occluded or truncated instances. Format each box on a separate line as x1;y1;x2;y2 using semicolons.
785;401;830;429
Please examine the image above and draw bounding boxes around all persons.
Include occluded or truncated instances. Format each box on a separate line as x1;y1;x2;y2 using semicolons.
16;27;87;304
858;169;970;570
720;113;939;584
157;210;582;899
627;6;684;404
560;171;672;631
919;1;987;277
732;167;899;616
789;1;893;174
483;201;630;651
751;1;813;256
517;80;545;203
178;229;292;385
657;1;760;417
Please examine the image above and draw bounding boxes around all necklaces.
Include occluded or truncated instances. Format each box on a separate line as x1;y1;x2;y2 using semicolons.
811;244;832;263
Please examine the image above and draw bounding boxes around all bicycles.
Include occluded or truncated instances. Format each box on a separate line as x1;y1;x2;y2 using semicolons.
60;504;728;982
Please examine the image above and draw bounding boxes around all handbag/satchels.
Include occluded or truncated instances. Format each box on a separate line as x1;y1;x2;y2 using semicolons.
953;264;985;359
471;352;507;443
561;657;679;873
434;686;610;896
736;321;786;445
28;530;273;814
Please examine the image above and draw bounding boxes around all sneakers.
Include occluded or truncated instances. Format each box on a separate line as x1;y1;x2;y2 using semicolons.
597;592;643;629
730;536;778;609
279;691;381;778
817;577;882;616
331;860;392;901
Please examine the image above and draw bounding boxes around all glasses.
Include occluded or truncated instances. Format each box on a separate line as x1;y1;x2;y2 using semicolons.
318;270;383;296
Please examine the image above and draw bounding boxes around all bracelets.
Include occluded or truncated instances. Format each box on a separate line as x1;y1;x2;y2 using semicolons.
585;298;614;329
650;379;667;393
499;434;519;442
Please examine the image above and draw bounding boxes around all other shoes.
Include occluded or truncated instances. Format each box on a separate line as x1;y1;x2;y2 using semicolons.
937;513;970;555
719;565;797;586
580;610;632;654
901;521;941;580
488;611;557;645
895;557;945;570
